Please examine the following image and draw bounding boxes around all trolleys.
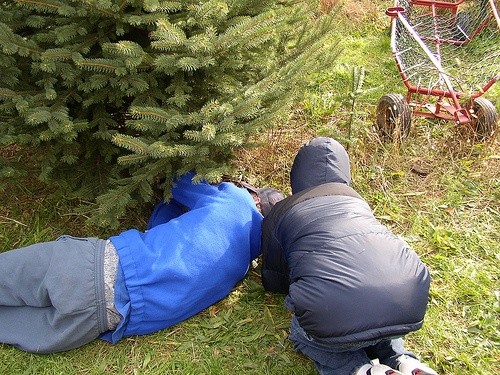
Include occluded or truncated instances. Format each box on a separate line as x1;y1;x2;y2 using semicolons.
376;0;500;142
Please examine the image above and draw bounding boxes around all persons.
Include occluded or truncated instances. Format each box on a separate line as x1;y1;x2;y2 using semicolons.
0;168;287;354
261;137;439;375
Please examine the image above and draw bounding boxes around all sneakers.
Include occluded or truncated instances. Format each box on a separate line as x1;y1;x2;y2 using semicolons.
353;355;440;375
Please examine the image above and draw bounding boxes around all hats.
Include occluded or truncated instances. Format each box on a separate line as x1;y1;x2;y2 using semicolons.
240;181;286;218
